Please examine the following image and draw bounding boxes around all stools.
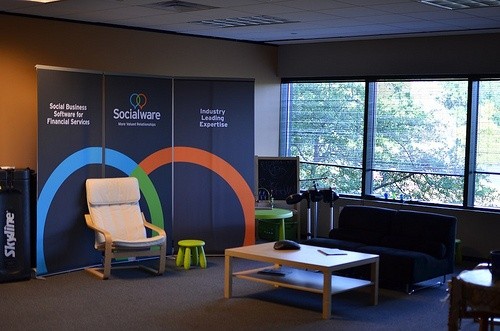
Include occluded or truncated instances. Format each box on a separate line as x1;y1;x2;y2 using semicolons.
176;239;207;270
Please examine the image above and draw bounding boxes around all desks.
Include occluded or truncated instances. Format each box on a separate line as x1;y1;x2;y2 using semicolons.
224;242;379;320
448;269;500;331
255;208;293;240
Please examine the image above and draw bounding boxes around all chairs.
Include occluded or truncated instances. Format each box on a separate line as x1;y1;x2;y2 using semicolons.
84;177;167;279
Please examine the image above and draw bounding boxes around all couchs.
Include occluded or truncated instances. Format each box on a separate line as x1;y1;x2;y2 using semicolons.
307;205;457;296
